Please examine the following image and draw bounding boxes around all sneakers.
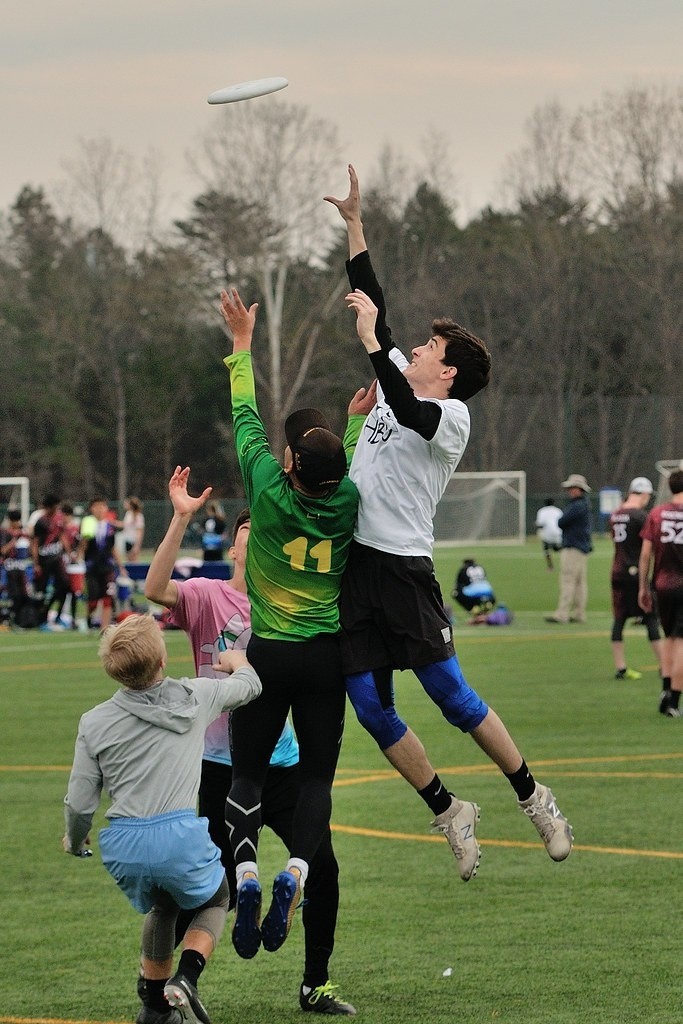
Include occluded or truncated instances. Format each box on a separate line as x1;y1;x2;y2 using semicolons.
233;879;262;960
300;981;356;1015
615;667;643;680
658;691;683;718
164;975;210;1024
135;1008;182;1024
429;792;481;884
517;782;574;862
262;867;300;952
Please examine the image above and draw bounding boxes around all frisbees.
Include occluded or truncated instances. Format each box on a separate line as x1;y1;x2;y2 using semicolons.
206;76;290;105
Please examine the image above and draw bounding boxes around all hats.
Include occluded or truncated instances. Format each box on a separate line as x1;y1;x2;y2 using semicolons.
284;408;347;491
629;476;662;499
560;474;591;494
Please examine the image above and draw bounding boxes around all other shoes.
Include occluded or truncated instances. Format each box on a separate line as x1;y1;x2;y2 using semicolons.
543;614;557;624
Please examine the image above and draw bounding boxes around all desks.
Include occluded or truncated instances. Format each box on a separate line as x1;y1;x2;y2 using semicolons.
127;563;228;582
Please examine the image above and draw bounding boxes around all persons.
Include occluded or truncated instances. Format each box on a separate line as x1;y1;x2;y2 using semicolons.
323;164;575;883
199;500;227;561
638;470;683;717
145;465;357;1015
0;494;144;633
62;614;262;1024
545;475;594;624
218;287;377;959
450;559;494;617
534;497;563;568
610;477;664;679
